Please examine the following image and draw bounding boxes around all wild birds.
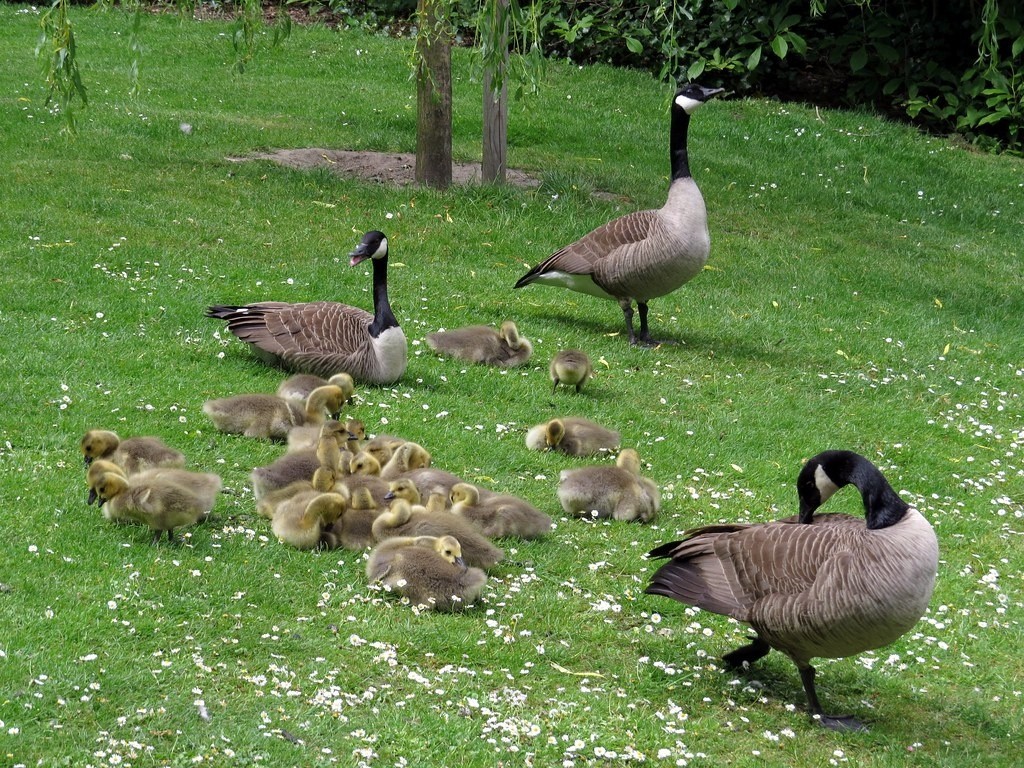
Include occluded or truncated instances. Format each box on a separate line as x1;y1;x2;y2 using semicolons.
201;228;408;387
524;415;619;458
549;348;595;398
204;371;552;614
78;428;223;547
423;321;534;368
514;82;726;348
640;449;939;729
554;449;662;525
